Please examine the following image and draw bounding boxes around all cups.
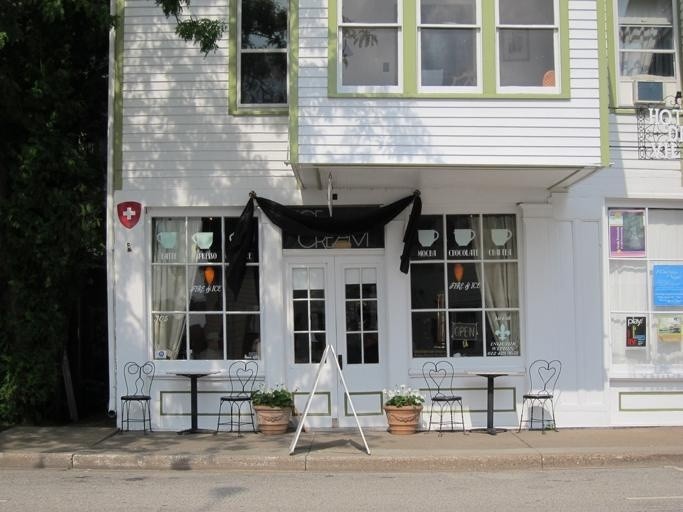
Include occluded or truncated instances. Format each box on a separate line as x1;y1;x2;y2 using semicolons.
416;228;440;248
490;227;514;247
453;228;476;247
156;231;178;250
190;232;216;251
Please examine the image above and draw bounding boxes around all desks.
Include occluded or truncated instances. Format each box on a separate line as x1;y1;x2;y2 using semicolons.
469;371;517;435
165;370;222;435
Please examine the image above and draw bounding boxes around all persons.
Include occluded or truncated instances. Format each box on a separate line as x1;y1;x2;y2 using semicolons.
671;317;679;324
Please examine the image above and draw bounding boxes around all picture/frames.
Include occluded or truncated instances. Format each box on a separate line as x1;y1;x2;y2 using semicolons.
497;27;530;62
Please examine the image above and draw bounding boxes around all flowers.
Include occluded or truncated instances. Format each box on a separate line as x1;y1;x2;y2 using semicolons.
382;383;425;407
249;382;302;407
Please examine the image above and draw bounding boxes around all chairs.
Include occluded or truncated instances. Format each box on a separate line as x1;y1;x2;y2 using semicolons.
516;358;562;435
120;360;156;435
420;359;466;438
215;360;259;438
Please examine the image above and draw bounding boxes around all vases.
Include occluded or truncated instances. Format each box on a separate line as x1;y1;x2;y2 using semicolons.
252;406;292;435
382;406;424;435
